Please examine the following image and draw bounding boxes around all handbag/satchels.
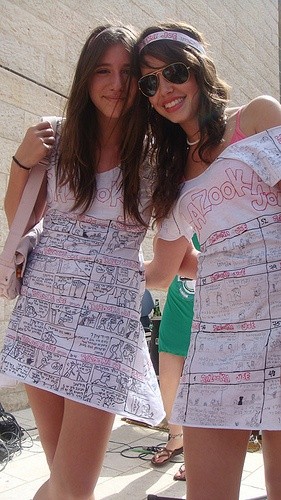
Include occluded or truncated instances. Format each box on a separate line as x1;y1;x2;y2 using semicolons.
0;116;57;300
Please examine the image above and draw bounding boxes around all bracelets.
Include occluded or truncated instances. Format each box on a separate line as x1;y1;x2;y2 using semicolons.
12;155;34;173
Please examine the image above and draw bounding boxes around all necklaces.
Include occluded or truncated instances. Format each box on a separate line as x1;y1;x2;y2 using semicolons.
186;137;200;145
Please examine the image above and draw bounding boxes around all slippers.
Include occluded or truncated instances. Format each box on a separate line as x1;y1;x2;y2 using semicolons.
151;447;183;466
174;463;186;481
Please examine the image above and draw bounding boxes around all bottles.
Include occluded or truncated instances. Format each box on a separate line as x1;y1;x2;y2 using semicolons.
153;298;161;317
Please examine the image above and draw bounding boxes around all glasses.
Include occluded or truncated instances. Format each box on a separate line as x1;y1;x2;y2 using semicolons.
137;62;191;98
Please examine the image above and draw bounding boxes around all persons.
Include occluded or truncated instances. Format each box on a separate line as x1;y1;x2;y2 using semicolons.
134;23;281;500
4;26;201;500
150;235;201;480
